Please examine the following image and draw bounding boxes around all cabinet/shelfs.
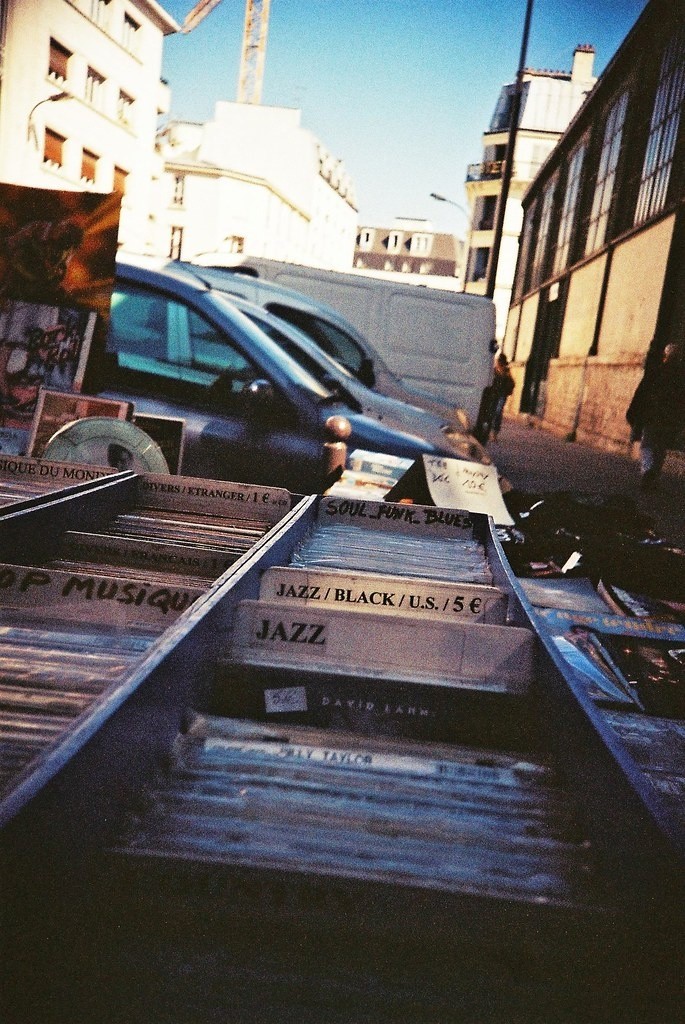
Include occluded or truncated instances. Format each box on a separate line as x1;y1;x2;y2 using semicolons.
1;451;685;1024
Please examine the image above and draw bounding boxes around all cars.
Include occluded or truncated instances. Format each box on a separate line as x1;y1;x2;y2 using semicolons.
110;259;496;518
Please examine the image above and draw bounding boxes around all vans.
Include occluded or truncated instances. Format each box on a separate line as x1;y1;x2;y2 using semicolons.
239;257;499;437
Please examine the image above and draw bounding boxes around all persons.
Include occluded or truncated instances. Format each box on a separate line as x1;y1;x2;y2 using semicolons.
624;344;685;490
483;347;516;442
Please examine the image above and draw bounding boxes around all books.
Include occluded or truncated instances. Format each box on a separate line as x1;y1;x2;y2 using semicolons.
0;476;685;1023
